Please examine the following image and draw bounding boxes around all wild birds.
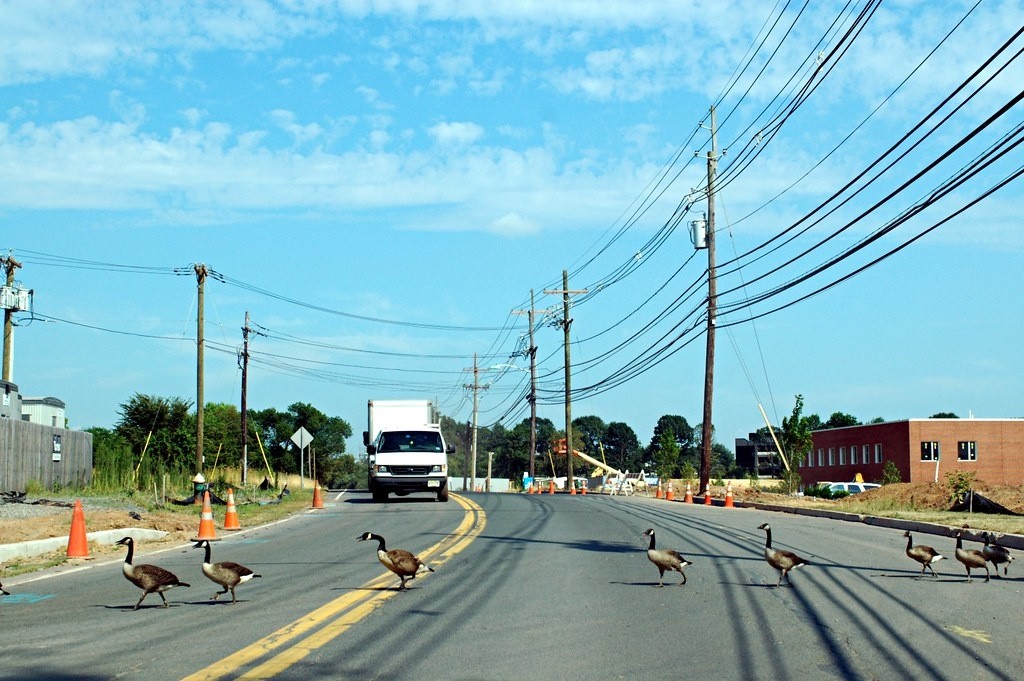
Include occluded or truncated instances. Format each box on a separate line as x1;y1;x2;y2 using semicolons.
356;532;435;591
116;537;191;612
193;539;261;604
757;523;811;588
903;530;948;580
980;531;1016;579
953;530;990;583
643;528;693;587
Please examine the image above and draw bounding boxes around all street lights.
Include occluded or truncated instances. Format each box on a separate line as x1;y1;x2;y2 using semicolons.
9;316;56;381
489;363;536;477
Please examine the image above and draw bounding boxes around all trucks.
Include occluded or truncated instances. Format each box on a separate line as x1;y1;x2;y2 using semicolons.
367;399;438;489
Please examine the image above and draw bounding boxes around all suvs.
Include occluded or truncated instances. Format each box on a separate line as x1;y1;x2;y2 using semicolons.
365;428;455;502
823;473;881;496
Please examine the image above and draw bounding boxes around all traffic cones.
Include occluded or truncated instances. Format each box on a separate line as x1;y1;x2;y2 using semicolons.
308;480;326;509
219;488;243;531
703;482;713;505
191;491;221;541
475;484;483;492
653;479;665;499
569;480;575;494
580;479;587;494
666;480;675;501
528;480;555;495
722;480;737;508
683;482;693;503
63;500;94;560
